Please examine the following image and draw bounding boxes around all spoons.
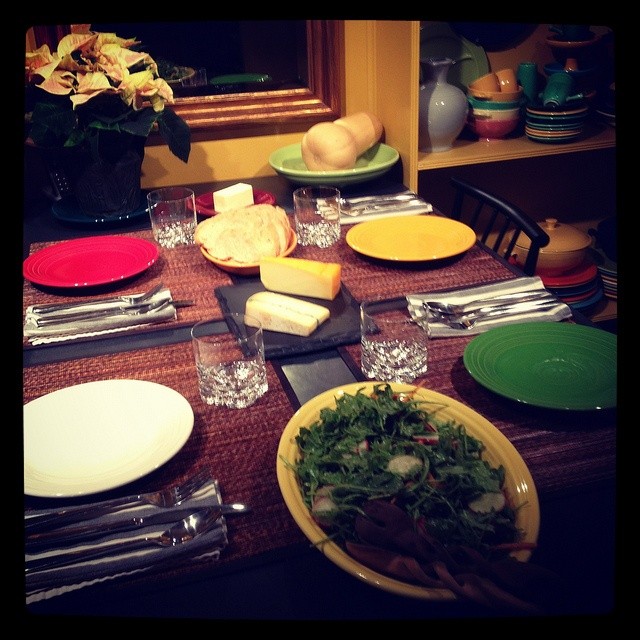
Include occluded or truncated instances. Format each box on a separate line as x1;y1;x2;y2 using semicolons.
425;293;556;315
25;505;222;575
335;203;428;216
37;298;171;328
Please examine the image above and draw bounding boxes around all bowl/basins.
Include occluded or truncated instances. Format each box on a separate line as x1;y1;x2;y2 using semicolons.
268;140;400;185
464;83;524;141
200;229;298;274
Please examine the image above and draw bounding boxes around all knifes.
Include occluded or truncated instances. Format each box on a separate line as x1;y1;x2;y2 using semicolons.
38;299;196;324
25;504;252;547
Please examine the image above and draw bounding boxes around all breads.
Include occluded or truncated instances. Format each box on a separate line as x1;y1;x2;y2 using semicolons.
213;182;254;211
194;203;292;267
260;255;342;299
245;291;330;338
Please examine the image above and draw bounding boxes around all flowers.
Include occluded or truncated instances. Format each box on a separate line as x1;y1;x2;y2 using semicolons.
27;32;190;165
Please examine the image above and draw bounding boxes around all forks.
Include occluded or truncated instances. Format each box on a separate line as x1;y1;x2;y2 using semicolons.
441;300;561;330
33;282;162;314
25;467;213;531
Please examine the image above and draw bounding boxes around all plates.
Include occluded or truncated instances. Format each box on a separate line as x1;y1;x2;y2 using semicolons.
346;216;477;261
463;321;617;411
507;254;604;309
23;235;161;289
23;379;195;499
49;187;154;223
189;189;276;217
524;104;591;144
596;261;617;299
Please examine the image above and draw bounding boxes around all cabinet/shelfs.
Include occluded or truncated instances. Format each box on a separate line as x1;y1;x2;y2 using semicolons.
374;21;617;323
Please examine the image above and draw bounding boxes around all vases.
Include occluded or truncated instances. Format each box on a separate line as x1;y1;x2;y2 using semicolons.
55;133;144;216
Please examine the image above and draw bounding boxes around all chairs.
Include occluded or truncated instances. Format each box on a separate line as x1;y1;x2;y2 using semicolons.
448;176;550;277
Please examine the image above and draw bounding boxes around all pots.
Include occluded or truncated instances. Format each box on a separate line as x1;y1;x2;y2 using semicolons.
508;218;592;273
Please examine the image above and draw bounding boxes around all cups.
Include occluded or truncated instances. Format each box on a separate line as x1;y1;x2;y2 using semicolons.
360;296;429;383
495;68;518;92
470;72;500;93
147;187;197;249
190;313;269;410
292;186;341;248
543;73;575;109
517;61;538;100
